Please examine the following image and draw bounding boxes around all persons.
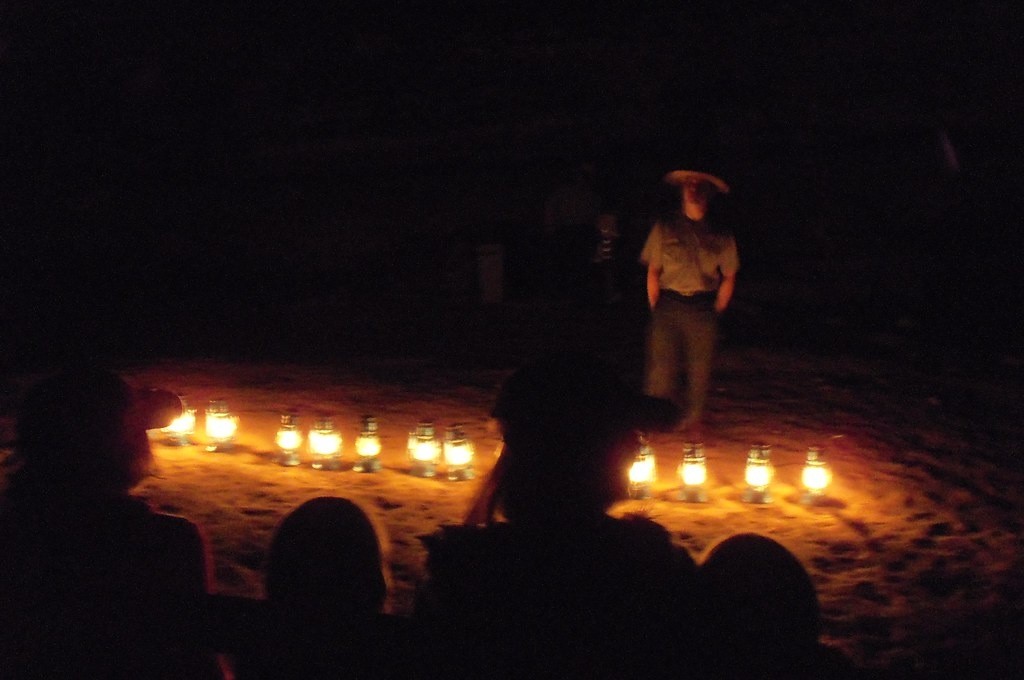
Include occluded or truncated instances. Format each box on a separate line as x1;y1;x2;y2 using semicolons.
638;170;738;431
546;171;623;304
0;369;210;680
700;534;860;680
240;497;433;680
416;357;705;680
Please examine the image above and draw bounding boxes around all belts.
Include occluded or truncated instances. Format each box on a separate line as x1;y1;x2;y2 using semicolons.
664;289;715;303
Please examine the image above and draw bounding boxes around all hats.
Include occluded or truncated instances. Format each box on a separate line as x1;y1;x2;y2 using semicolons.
666;162;729;195
